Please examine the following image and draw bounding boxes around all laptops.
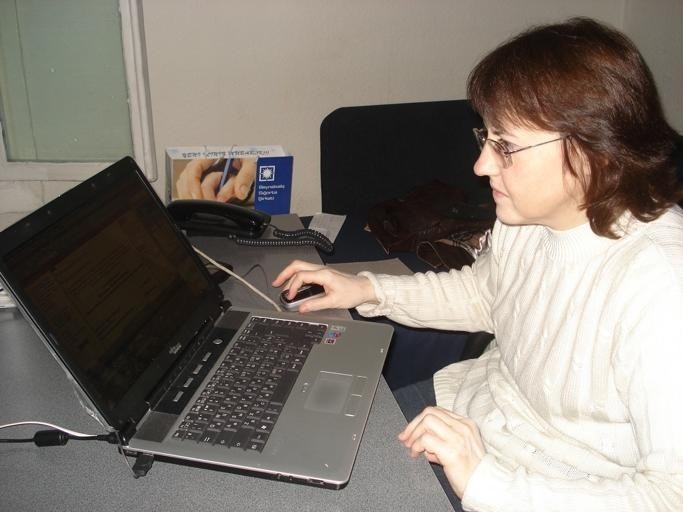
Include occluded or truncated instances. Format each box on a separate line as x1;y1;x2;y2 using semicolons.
0;156;395;490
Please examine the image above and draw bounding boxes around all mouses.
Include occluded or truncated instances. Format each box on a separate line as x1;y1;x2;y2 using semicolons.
280;284;323;309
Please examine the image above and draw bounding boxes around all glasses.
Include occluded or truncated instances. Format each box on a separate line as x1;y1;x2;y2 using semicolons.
473;128;571;169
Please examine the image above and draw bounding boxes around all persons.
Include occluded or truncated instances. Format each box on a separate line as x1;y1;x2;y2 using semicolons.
173;158;259;207
268;15;682;512
197;171;255;206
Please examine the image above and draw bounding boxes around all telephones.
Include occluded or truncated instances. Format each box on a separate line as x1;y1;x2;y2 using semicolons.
166;200;271;240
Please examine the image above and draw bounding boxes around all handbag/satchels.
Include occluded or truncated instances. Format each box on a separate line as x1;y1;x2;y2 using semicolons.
369;187;496;252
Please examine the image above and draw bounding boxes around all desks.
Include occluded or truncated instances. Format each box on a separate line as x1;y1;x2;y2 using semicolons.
0;206;458;512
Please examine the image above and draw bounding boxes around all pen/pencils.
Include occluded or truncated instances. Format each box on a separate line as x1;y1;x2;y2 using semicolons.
219;158;231;192
